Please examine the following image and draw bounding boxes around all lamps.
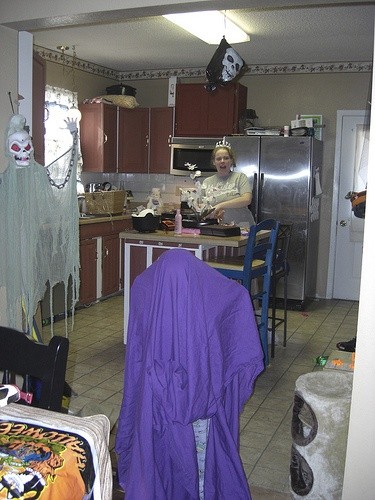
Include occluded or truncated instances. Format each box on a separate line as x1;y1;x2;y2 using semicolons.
162;10;250;44
67;44;82;122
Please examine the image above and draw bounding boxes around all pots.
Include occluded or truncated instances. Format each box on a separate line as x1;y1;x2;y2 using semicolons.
106;83;137;97
132;214;159;233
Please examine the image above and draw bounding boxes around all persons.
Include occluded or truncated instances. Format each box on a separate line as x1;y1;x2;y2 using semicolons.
336;186;367;352
199;136;264;319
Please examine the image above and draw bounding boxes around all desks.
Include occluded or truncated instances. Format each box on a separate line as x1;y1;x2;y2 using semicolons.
0;402;113;500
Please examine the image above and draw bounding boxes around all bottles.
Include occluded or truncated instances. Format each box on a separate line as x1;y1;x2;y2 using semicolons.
175;209;182;237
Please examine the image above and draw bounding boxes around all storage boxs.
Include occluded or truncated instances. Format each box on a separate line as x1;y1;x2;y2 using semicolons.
168;76;181;107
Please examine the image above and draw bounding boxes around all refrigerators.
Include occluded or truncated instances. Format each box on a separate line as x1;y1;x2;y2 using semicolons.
226;136;323;310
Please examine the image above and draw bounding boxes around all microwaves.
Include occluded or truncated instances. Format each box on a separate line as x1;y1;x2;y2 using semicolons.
169;144;218;177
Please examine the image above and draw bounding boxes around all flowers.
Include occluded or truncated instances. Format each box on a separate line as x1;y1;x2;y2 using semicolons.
178;162;240;220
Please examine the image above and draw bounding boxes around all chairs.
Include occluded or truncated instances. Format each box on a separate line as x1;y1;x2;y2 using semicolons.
0;326;69;412
255;223;294;358
202;219;280;365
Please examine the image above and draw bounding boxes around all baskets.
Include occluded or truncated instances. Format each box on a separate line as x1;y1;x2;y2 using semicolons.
83;190;126;215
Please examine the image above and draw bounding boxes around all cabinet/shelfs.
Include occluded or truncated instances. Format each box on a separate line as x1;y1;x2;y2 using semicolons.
175;83;247;138
79;103;118;173
119;107;173;174
80;219;133;307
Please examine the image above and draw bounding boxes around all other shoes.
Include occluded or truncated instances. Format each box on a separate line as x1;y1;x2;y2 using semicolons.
336;336;356;352
